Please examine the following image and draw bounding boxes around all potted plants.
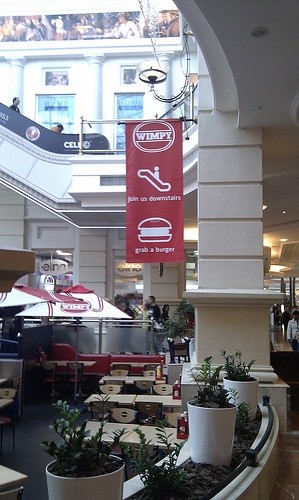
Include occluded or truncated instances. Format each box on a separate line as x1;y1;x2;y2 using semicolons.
186;356;238;471
40;399;125;500
222;349;260;422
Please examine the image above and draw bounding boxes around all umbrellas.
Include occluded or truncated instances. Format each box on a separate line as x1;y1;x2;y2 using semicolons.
0;283;84;339
14;284;133;408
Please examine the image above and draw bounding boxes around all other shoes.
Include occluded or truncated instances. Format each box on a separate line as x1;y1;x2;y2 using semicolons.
162;348;169;352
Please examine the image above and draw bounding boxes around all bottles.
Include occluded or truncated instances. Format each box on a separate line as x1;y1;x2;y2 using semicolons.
173;379;181;399
160;356;164;364
156;365;161;380
177;409;188;439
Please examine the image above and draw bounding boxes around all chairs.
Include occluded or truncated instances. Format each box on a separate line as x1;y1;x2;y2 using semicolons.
99;362;174;423
1;388;17;450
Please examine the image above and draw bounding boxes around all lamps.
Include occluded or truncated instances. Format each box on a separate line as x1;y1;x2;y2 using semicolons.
137;25;194;104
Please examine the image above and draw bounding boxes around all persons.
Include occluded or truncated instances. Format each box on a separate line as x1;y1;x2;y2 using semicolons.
115;294;170;355
51;124;63;133
0;9;181;43
271;305;299;369
9;97;20;114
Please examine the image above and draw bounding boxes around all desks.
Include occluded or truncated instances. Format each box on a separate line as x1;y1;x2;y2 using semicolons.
132;395;173;422
84;394;137;419
66;422;139;458
99;376;156;392
122;426;188;451
